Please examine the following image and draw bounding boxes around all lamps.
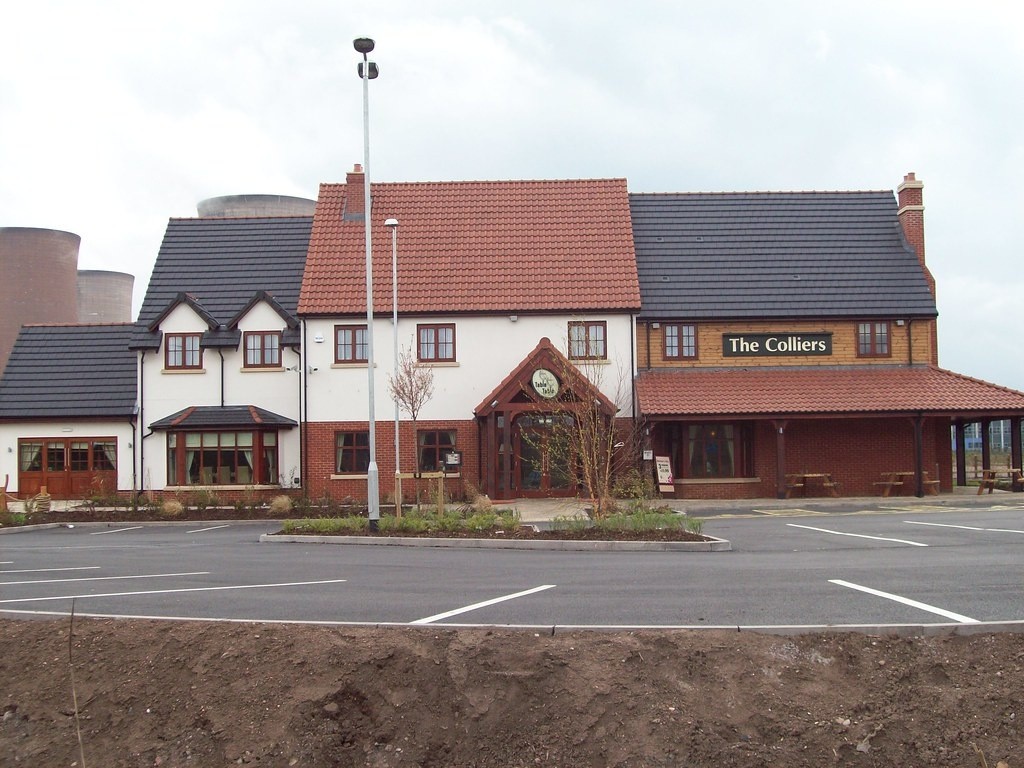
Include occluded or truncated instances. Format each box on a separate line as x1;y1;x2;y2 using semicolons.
653;323;659;328
314;336;324;343
510;316;517;321
897;320;904;325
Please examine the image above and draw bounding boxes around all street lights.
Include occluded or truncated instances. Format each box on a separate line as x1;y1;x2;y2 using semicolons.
354;36;379;531
384;218;402;517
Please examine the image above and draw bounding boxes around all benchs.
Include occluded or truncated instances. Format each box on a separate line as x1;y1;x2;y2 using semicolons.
968;478;1024;483
823;482;838;487
787;483;804;487
876;482;903;485
923;480;940;484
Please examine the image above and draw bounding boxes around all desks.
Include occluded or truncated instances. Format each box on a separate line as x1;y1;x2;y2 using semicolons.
881;471;937;497
786;474;831;477
973;468;1024;495
